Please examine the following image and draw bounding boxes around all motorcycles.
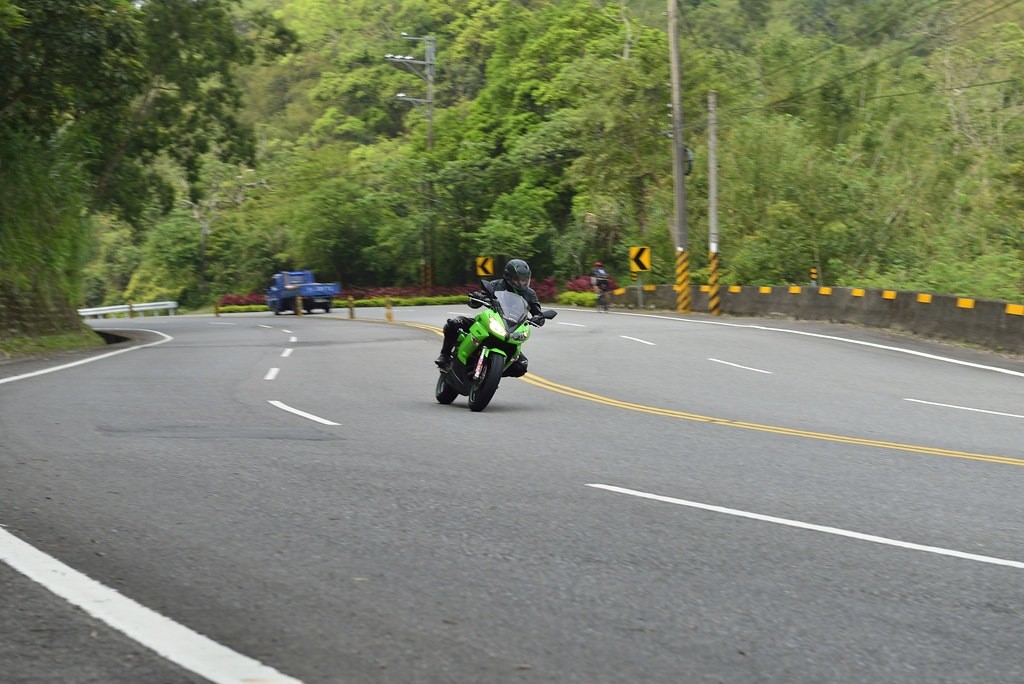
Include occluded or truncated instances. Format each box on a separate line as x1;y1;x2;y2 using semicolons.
435;279;558;412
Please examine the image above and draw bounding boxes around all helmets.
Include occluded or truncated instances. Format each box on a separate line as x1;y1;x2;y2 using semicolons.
504;259;531;291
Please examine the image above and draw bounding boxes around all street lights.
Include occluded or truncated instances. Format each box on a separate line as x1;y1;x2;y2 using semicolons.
384;31;441;299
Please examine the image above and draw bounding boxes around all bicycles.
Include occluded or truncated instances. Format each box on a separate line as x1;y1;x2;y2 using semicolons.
593;282;610;310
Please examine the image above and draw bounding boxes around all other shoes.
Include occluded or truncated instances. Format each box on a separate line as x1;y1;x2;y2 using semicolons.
435;353;449;367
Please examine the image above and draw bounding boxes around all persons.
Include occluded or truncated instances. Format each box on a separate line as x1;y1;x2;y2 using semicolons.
591;262;610;299
435;259;545;377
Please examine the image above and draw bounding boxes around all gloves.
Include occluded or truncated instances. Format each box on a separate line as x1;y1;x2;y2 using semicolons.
533;315;544;327
472;291;485;305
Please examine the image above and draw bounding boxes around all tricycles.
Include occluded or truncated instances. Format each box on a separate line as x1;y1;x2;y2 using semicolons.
265;271;341;315
